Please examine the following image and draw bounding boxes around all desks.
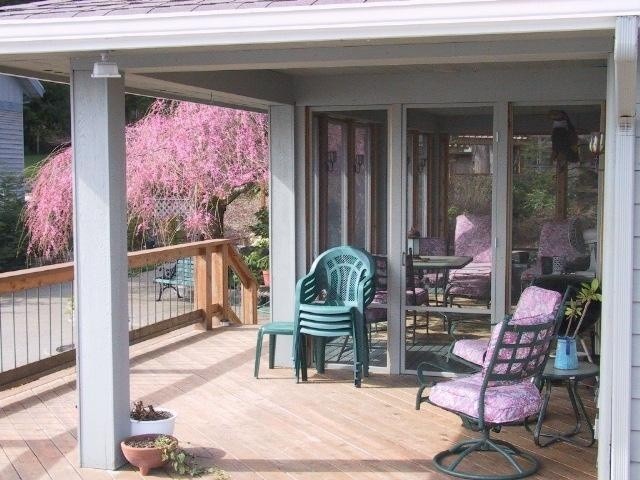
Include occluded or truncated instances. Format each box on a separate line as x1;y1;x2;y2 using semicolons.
522;354;603;450
409;255;473;335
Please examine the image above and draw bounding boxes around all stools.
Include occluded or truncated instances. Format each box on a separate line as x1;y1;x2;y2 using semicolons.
252;320;310;383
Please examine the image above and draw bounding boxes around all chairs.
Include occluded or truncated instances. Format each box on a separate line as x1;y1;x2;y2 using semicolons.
414;286;566;480
447;212;592;309
290;247;430;389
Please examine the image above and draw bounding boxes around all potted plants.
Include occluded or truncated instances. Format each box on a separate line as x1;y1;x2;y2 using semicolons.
128;399;177;439
241;206;270;287
120;434;232;479
554;278;601;369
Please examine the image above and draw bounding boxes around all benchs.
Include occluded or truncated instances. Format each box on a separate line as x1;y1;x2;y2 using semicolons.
151;258;195;302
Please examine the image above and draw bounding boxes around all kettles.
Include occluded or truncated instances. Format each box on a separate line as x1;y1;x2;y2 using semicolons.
553;336;580;370
